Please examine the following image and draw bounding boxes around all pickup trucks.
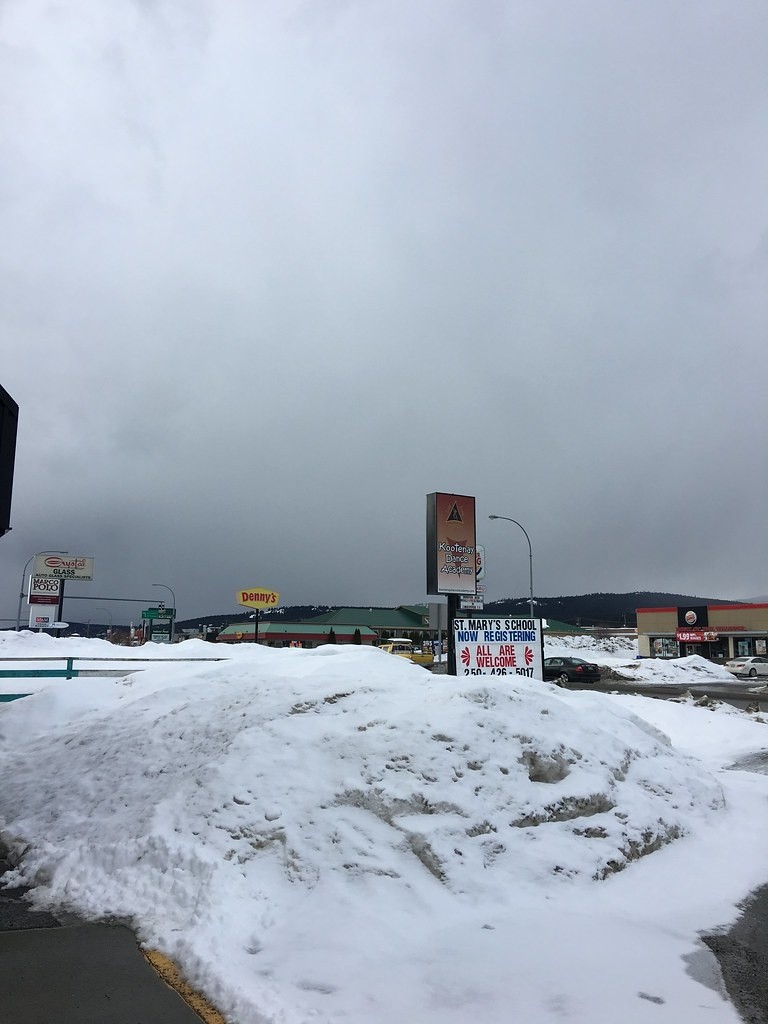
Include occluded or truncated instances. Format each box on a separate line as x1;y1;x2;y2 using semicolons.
377;643;435;670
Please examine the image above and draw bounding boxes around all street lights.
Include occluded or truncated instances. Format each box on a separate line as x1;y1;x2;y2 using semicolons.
489;514;534;620
15;551;69;632
152;584;175;643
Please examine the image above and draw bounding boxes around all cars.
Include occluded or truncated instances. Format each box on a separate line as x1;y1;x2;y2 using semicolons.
543;656;602;685
725;657;768;677
